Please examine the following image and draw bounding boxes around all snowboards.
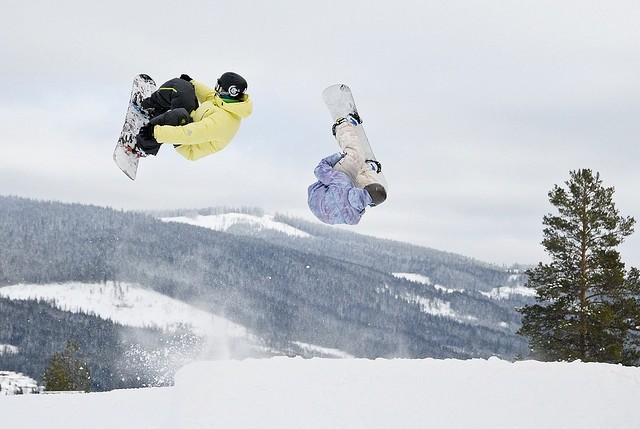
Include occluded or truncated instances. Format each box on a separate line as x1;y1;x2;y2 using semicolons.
114;73;156;180
323;85;391;207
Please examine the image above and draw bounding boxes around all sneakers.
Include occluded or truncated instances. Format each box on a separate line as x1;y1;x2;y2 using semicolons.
367;160;381;174
332;114;361;137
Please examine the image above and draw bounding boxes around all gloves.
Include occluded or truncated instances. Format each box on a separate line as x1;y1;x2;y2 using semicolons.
180;74;193;81
139;122;155;141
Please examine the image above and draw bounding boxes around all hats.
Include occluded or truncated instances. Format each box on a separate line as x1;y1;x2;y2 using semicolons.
218;72;248;100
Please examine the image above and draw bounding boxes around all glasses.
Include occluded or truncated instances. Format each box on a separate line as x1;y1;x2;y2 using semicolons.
215;84;224;92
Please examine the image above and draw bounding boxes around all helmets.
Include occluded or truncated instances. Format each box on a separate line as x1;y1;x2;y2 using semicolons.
364;183;387;207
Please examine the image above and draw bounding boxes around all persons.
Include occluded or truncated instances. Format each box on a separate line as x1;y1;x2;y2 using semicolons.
307;113;388;226
123;71;253;162
